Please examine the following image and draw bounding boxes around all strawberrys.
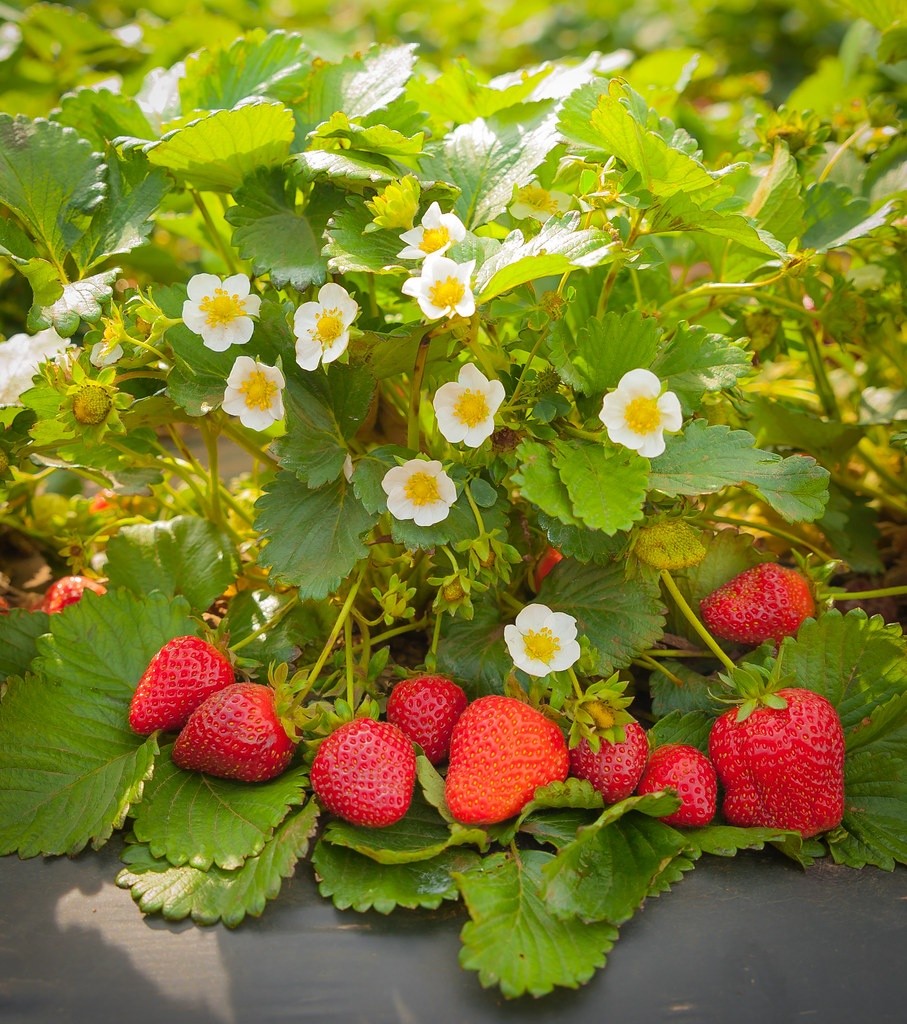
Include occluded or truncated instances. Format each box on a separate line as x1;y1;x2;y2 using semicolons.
0;543;846;842
